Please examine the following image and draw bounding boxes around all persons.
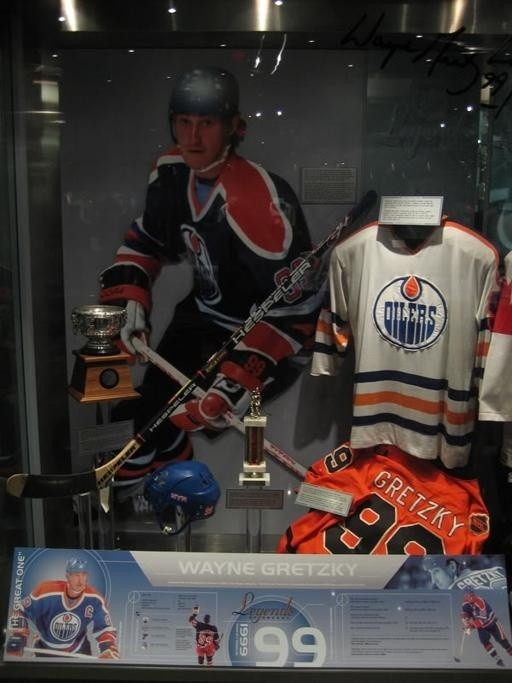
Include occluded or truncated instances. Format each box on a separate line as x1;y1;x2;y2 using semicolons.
249;385;263;418
92;65;327;490
460;585;512;668
6;558;120;660
188;604;220;666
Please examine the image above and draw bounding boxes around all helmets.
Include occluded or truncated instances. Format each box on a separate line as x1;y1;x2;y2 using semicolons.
66;558;90;575
168;66;241;118
144;458;221;533
463;585;473;594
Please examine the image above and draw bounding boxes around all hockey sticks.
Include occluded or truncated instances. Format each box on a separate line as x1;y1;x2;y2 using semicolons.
7;189;378;499
454;628;467;662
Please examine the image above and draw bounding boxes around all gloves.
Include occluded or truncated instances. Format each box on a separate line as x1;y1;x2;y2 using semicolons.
102;301;151;365
169;363;262;433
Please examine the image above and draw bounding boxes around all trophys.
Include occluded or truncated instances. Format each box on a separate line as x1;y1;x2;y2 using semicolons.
238;385;271;489
